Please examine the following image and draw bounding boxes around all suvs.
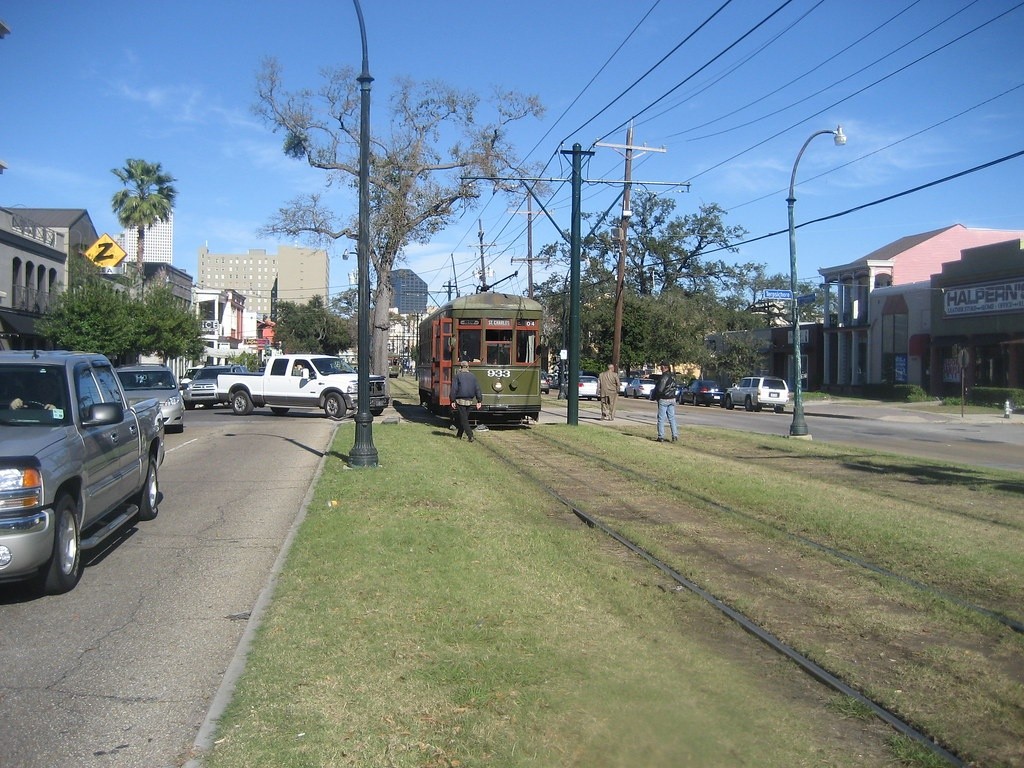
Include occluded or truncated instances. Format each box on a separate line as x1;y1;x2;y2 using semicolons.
115;362;185;433
177;363;249;410
723;376;789;414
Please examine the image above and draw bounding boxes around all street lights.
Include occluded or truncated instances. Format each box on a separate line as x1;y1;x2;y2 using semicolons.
787;129;850;439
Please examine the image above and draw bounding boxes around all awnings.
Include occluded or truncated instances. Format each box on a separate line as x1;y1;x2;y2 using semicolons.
0;311;50;340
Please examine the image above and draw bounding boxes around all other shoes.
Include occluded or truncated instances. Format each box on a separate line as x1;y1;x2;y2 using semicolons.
671;437;676;443
469;437;474;442
657;437;663;442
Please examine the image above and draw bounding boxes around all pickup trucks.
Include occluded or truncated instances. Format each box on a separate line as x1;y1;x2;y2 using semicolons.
0;348;167;594
215;354;390;418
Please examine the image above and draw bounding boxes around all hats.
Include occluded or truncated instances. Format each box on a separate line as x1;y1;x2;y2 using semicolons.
461;361;468;367
657;360;669;366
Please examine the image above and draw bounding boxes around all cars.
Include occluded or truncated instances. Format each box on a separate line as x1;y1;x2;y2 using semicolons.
561;375;601;402
677;379;726;407
623;377;656;400
616;376;635;396
541;371;559;394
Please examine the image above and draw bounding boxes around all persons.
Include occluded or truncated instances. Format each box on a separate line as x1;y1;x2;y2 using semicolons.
449;361;482;442
292;363;305;376
598;364;620;420
9;374;58;419
652;360;679;442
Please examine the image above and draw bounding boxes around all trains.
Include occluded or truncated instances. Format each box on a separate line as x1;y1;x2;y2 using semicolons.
415;292;545;426
